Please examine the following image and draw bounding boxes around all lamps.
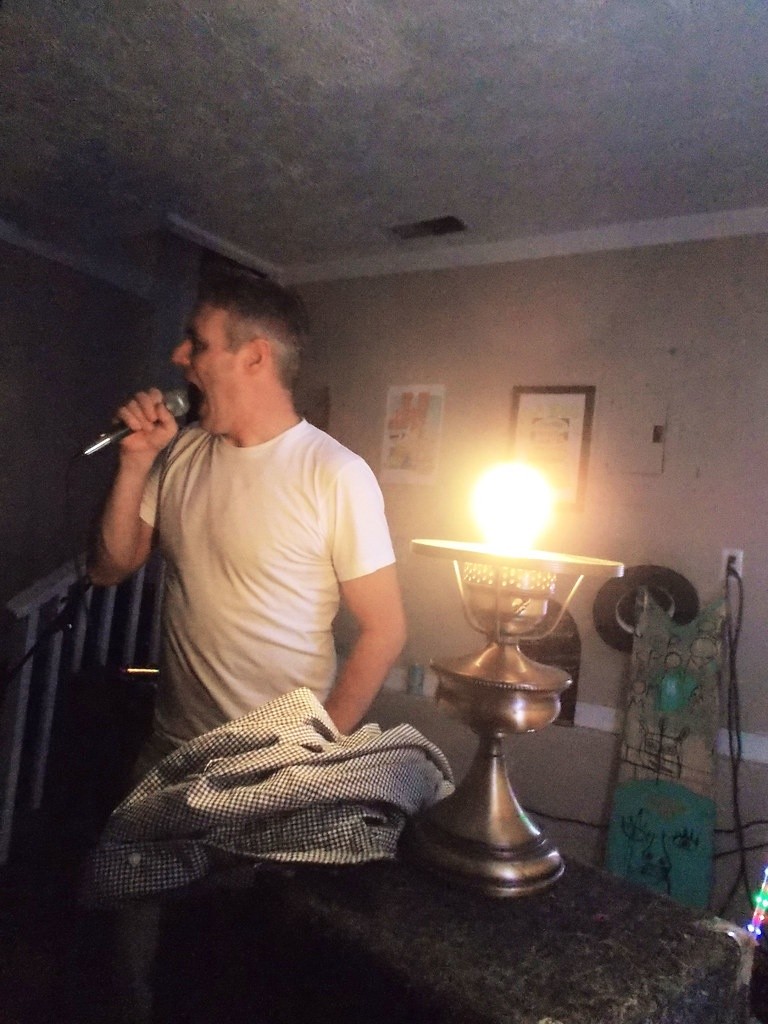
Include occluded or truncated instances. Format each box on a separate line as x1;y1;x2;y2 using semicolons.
392;462;623;897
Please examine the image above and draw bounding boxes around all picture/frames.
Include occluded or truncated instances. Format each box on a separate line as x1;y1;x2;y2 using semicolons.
381;383;446;486
510;385;596;483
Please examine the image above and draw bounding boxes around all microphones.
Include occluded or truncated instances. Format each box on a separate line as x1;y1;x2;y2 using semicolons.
73;385;190;462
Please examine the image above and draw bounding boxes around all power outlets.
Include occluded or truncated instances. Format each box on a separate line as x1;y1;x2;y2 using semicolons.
721;548;742;581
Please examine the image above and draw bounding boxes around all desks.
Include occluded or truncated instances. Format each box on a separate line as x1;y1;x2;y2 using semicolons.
167;801;756;1024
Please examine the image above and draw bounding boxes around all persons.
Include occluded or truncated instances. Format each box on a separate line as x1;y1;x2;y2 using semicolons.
86;265;408;815
